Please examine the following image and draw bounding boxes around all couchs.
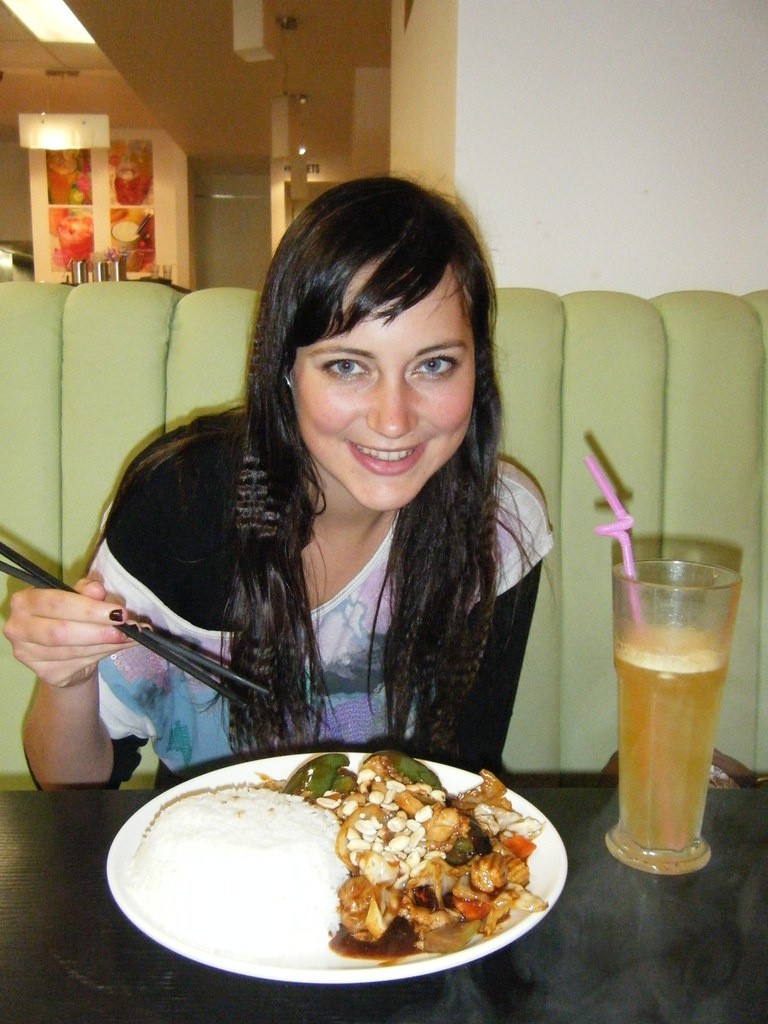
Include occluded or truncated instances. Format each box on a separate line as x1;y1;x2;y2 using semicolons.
0;275;768;792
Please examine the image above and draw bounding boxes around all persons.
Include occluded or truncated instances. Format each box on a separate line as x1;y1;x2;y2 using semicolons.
3;178;555;795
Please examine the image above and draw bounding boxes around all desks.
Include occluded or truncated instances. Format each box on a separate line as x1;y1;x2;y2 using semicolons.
0;791;768;1024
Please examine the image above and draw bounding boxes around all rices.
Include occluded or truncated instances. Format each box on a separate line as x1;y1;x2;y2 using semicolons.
127;786;349;963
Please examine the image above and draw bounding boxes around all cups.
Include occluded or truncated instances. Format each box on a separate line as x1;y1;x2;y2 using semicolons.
70;258;124;286
604;558;742;874
46;150;149;271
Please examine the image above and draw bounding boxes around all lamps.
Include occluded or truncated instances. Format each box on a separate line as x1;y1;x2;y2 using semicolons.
19;68;111;152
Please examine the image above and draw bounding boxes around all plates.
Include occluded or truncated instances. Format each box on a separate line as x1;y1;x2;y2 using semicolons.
106;752;570;984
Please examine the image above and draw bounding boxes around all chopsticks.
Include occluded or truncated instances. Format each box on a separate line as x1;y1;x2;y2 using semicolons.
0;542;271;710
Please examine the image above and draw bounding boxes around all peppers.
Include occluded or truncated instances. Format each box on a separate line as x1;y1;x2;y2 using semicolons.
363;750;444;791
283;753;348;802
